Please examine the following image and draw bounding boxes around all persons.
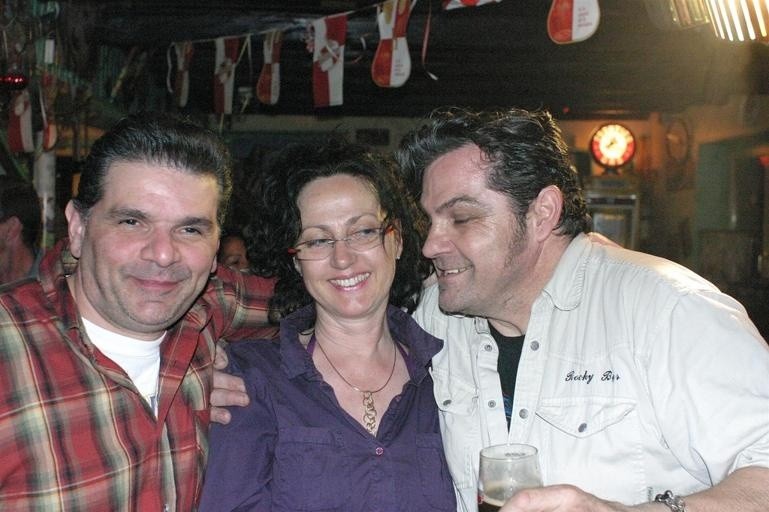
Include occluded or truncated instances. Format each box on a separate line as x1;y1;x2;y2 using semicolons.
217;228;250;275
1;111;287;510
209;106;769;512
0;171;46;288
197;143;458;512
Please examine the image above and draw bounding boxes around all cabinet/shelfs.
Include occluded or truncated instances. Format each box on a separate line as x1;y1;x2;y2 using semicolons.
583;173;640;251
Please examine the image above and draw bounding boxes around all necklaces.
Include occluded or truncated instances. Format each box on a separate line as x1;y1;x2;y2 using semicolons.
313;328;397;434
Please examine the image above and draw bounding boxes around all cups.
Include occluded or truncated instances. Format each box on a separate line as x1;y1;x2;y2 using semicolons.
477;443;543;512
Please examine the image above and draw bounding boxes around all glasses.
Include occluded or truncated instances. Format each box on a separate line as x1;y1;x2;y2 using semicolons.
286;223;396;261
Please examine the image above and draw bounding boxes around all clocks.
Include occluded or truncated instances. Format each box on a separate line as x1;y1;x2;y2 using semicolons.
666;116;691;167
588;121;637;169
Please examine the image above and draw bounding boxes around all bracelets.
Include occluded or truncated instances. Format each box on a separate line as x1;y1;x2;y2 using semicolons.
655;489;686;511
